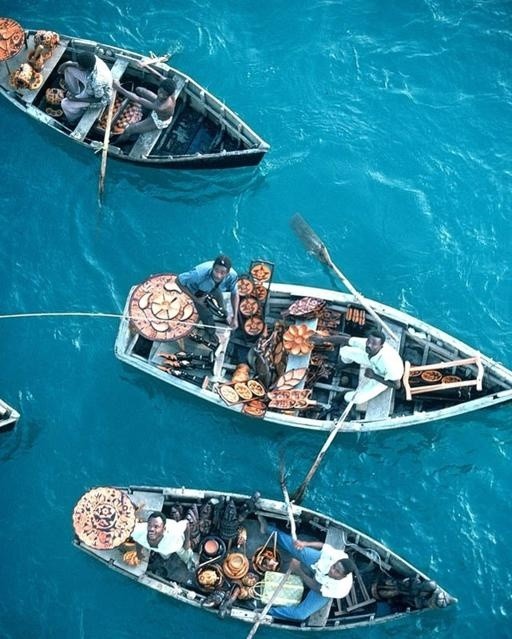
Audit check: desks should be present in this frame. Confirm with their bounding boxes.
[128,273,201,350]
[0,17,28,74]
[72,487,137,550]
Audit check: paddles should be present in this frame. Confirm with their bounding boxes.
[292,392,359,507]
[279,467,299,543]
[289,213,398,342]
[99,88,118,193]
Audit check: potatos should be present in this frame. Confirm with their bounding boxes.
[100,93,143,133]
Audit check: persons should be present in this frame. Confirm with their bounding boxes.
[127,504,196,573]
[176,254,239,344]
[259,514,354,621]
[109,79,176,145]
[57,51,113,125]
[324,331,404,406]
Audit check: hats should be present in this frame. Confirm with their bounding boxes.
[215,255,231,269]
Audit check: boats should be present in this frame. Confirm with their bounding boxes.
[72,487,459,632]
[114,284,512,432]
[0,29,271,170]
